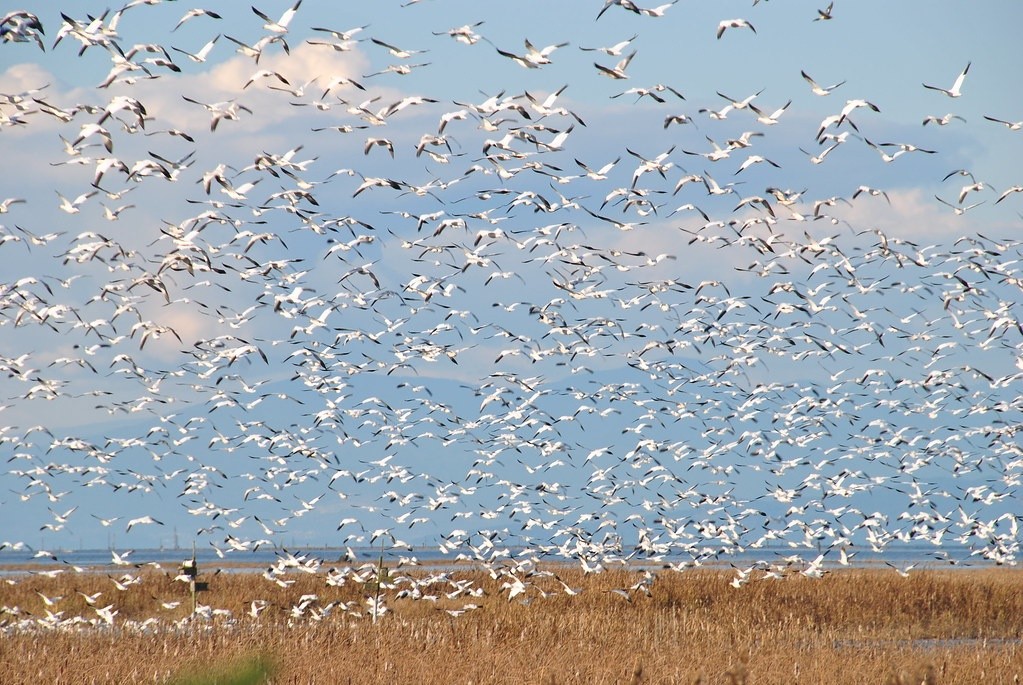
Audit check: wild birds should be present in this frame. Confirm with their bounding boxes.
[0,0,1023,635]
